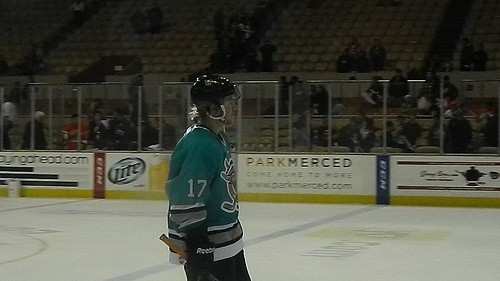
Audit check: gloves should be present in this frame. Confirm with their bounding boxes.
[186,224,214,272]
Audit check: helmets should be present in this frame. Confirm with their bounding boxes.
[190,75,241,110]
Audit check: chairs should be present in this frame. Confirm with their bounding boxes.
[0,0,500,154]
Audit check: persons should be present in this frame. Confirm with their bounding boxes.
[0,0,499,152]
[165,75,252,281]
